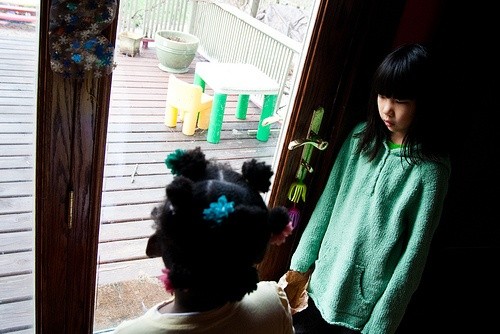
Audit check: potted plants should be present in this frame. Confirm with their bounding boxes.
[118,7,146,58]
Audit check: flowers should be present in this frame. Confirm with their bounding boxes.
[270,221,294,247]
[202,195,237,223]
[165,149,190,180]
[158,268,177,294]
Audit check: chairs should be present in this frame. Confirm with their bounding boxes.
[164,73,215,136]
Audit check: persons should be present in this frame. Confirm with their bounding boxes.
[111,146,296,334]
[289,44,453,334]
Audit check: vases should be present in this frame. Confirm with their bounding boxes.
[154,30,200,74]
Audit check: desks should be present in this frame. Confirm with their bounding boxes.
[194,62,280,143]
